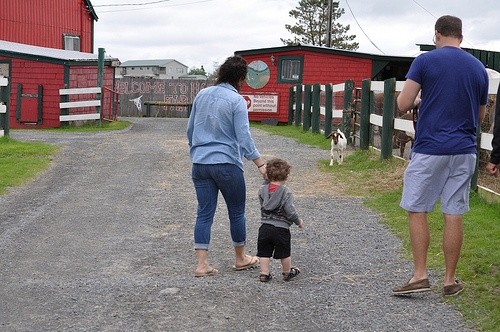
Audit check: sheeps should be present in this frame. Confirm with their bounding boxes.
[325,128,348,168]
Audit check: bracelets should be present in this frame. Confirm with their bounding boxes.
[258,164,264,168]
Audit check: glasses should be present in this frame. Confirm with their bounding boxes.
[433,35,437,44]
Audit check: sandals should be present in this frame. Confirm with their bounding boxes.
[259,272,272,282]
[282,267,301,281]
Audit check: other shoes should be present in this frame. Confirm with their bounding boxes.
[444,279,464,297]
[391,276,431,294]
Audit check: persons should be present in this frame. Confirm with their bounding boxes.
[258,158,304,282]
[187,55,271,277]
[486,82,500,176]
[392,15,489,297]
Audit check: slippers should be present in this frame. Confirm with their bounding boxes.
[194,267,218,277]
[236,256,260,270]
